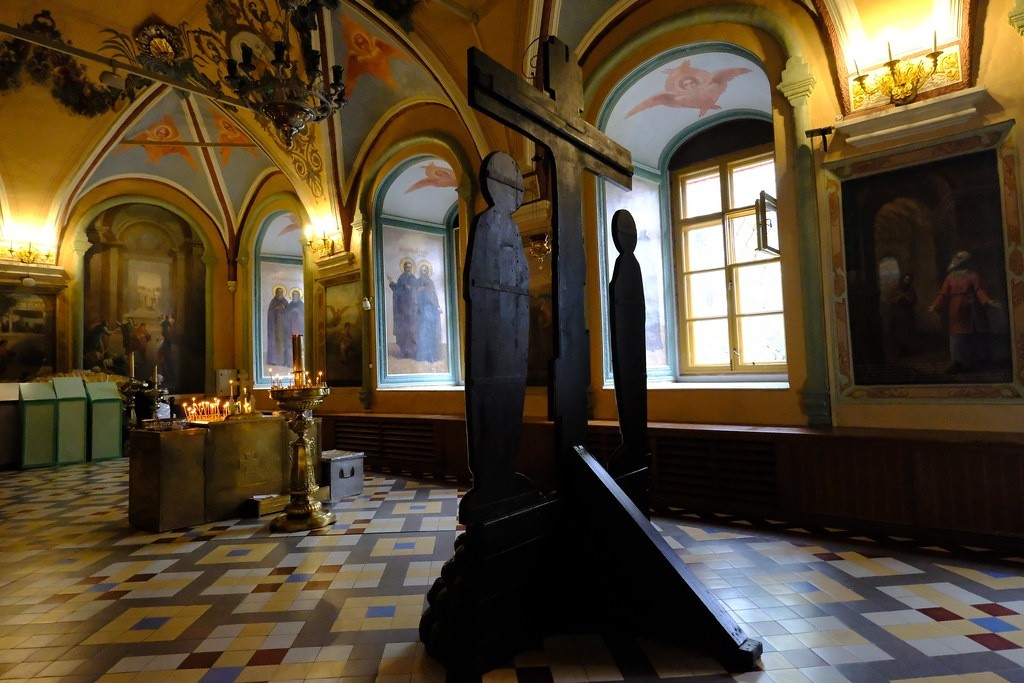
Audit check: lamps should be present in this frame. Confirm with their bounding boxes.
[852,29,944,105]
[8,239,51,264]
[224,0,349,149]
[305,214,329,251]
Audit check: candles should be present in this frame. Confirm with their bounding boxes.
[269,367,323,388]
[230,379,234,396]
[152,364,158,383]
[244,388,248,413]
[129,351,135,377]
[182,397,242,421]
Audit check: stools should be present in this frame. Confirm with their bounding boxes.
[321,449,366,501]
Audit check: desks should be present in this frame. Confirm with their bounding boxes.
[127,427,207,530]
[181,415,323,523]
